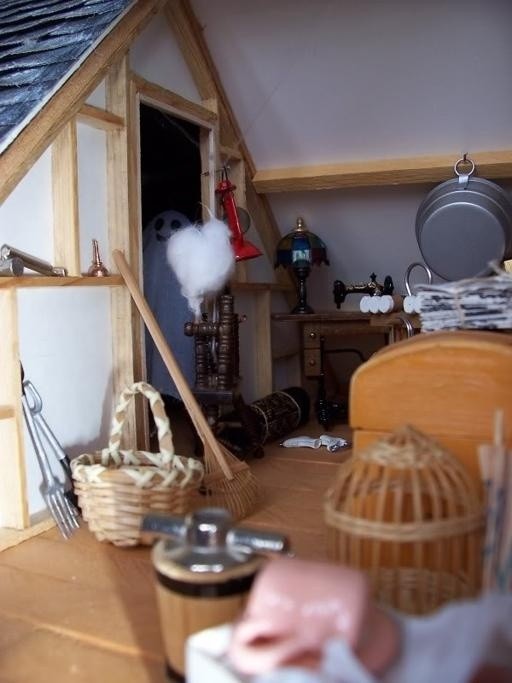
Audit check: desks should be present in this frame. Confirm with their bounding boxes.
[271,312,421,378]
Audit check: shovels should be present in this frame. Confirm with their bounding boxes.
[23,379,82,515]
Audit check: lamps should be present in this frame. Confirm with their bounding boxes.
[273,218,331,314]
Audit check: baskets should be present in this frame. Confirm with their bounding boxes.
[67,382,207,549]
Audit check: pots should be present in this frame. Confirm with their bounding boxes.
[416,157,512,284]
